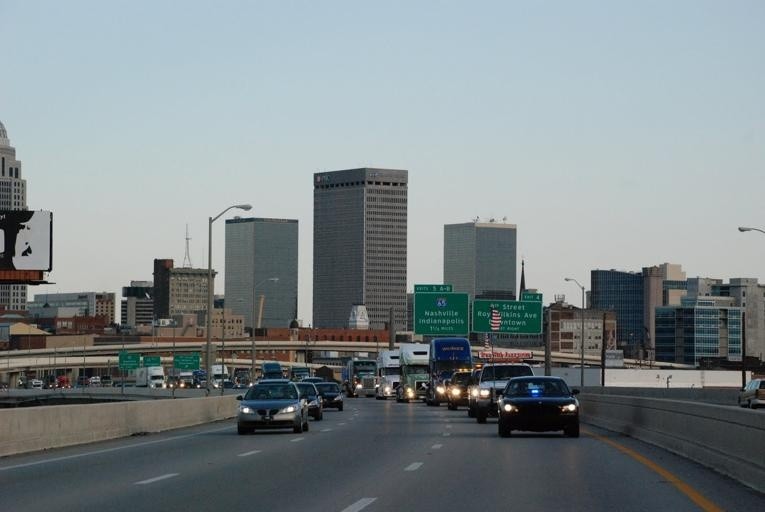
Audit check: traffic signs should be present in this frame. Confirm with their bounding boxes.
[472,290,545,335]
[142,356,161,367]
[413,283,471,338]
[174,354,201,370]
[119,353,141,369]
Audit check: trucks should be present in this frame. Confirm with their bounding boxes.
[347,337,473,408]
[17,364,229,390]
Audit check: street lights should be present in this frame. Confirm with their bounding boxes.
[206,204,252,394]
[565,277,586,388]
[249,277,282,339]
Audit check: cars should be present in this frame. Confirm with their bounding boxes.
[229,361,343,436]
[443,362,581,441]
[737,379,765,409]
